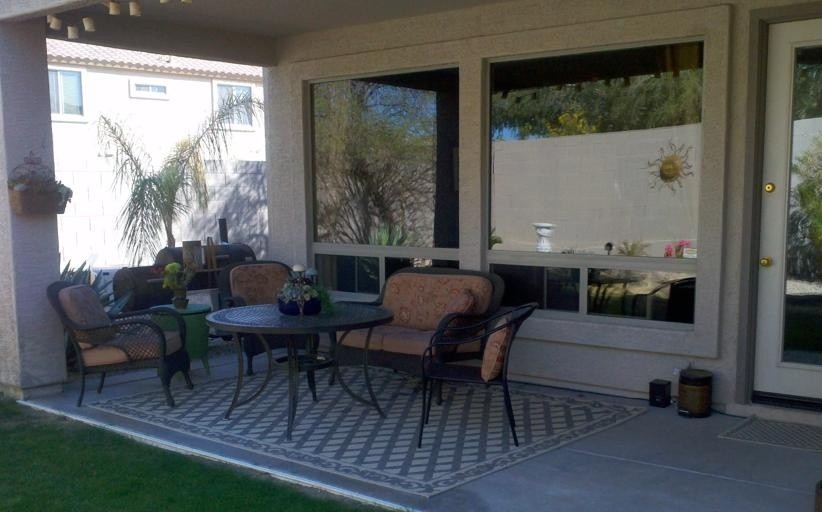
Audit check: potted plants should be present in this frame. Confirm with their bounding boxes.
[6,164,71,217]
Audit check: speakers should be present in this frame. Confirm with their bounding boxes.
[649,379,671,408]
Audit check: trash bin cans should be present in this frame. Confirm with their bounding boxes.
[677,368,713,418]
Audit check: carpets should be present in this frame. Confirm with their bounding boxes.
[714,416,822,454]
[16,343,658,502]
